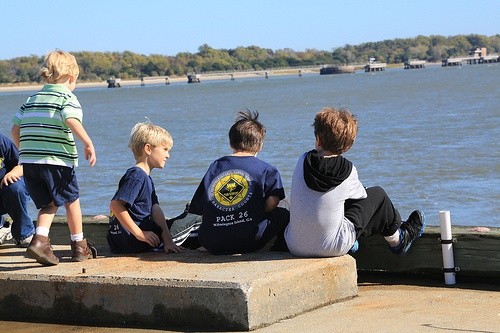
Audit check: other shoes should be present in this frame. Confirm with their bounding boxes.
[16,234,33,247]
[0,223,13,245]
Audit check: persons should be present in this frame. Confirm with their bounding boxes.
[0,133,35,249]
[107,122,201,255]
[283,107,427,258]
[188,109,290,256]
[10,51,97,267]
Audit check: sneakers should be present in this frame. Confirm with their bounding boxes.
[27,233,59,266]
[388,209,426,256]
[71,238,97,262]
